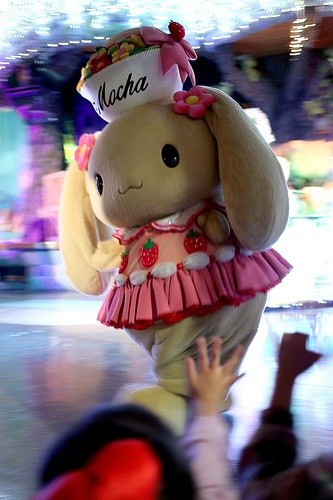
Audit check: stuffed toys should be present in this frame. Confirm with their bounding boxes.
[55,19,292,439]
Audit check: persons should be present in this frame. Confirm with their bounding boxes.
[233,331,331,499]
[27,334,240,500]
